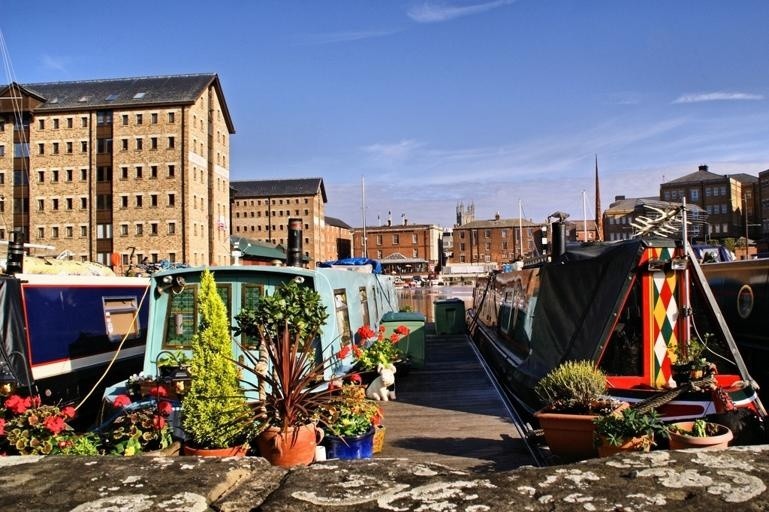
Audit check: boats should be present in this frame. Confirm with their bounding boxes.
[102,258,398,410]
[1,226,153,437]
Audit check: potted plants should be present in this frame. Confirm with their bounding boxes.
[184,268,385,470]
[533,333,734,458]
[154,350,193,377]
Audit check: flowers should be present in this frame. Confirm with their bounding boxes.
[0,384,181,456]
[352,324,416,368]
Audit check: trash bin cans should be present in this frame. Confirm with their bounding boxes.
[433,299,467,334]
[379,311,426,370]
